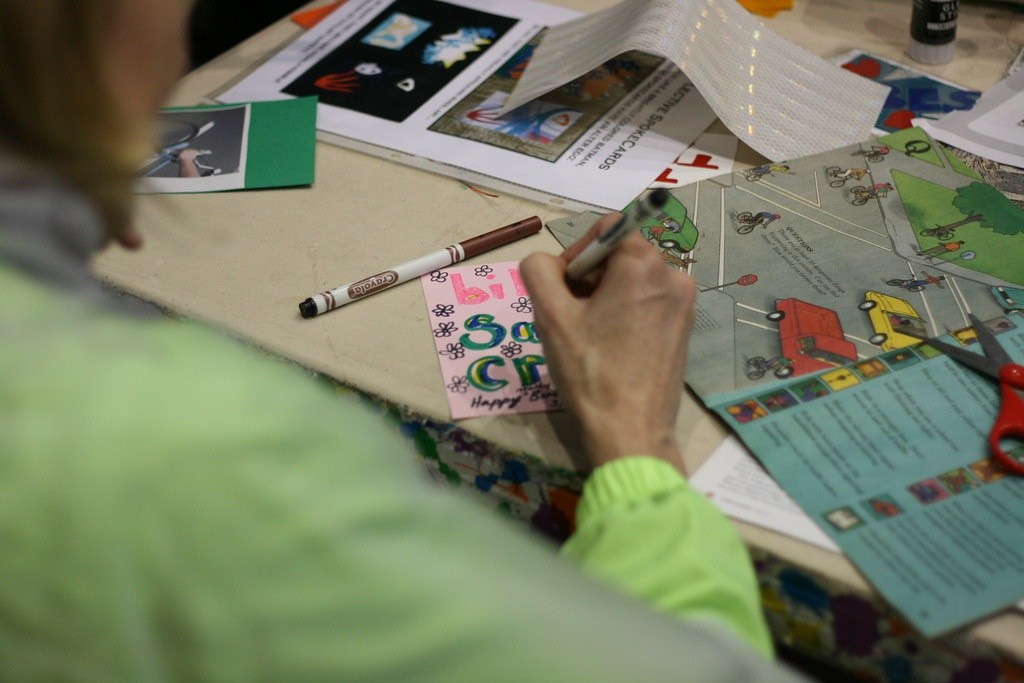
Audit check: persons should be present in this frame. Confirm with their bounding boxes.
[178,148,201,178]
[0,0,804,682]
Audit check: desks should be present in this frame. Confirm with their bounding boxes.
[80,0,1024,683]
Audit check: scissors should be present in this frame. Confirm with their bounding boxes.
[923,309,1024,479]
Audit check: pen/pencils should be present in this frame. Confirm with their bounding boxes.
[299,212,544,327]
[565,190,673,287]
